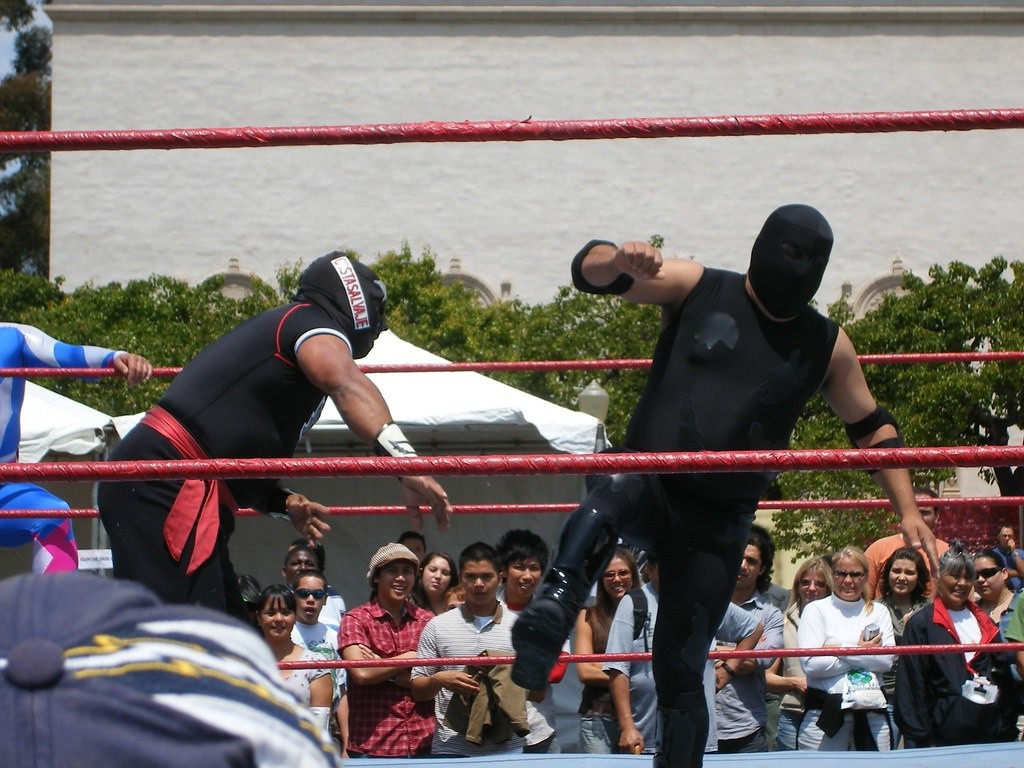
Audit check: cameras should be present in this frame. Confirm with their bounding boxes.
[864,623,879,642]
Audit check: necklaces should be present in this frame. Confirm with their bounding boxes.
[984,590,1012,615]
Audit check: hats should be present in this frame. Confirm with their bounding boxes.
[366,542,420,587]
[1,570,343,768]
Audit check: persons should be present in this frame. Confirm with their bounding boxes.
[97,251,450,621]
[232,512,1024,758]
[0,323,152,576]
[0,574,339,768]
[510,204,943,768]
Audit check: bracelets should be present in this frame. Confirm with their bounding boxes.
[722,662,735,677]
[1012,552,1019,559]
[372,421,419,482]
[270,485,293,513]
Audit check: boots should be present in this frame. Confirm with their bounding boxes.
[512,508,617,691]
[653,703,710,768]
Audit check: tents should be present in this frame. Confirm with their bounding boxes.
[19,329,614,612]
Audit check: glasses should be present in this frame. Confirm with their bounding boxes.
[974,567,1000,579]
[832,569,866,580]
[797,578,827,589]
[294,589,326,600]
[602,569,633,583]
